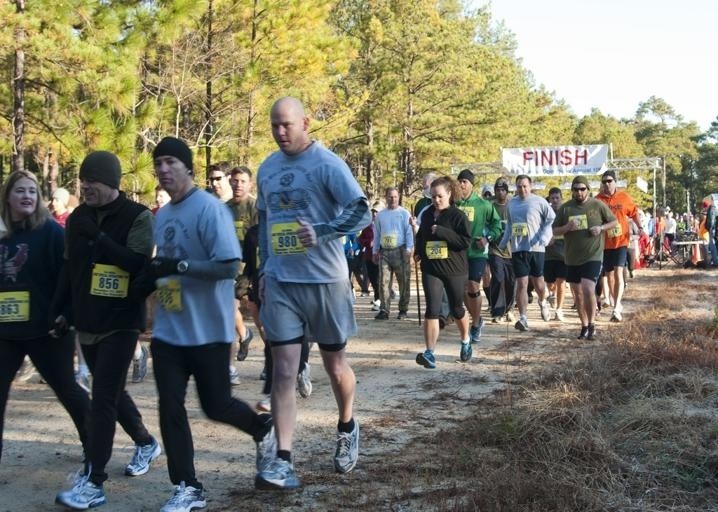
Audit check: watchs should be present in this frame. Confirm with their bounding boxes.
[177,259,191,275]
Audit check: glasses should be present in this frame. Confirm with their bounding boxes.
[209,176,222,180]
[573,188,586,192]
[602,179,613,184]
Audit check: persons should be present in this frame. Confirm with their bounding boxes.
[243,224,311,411]
[50,151,161,509]
[131,341,149,382]
[544,187,567,321]
[360,200,384,311]
[552,177,618,340]
[344,230,368,298]
[206,162,241,385]
[49,188,70,226]
[1,171,91,481]
[254,97,373,490]
[224,167,259,361]
[416,176,472,369]
[373,186,413,319]
[646,201,718,267]
[152,137,276,512]
[499,174,555,331]
[594,171,646,322]
[485,177,515,323]
[456,169,503,343]
[153,186,170,212]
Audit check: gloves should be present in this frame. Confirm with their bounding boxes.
[149,256,180,278]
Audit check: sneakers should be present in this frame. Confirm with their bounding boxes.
[255,424,278,471]
[361,292,369,296]
[236,329,253,360]
[375,311,389,320]
[416,350,436,369]
[587,323,595,340]
[55,478,105,509]
[125,438,161,475]
[297,362,312,399]
[470,325,480,342]
[132,345,148,382]
[160,482,207,512]
[549,297,556,308]
[515,318,528,331]
[255,459,301,491]
[333,418,360,474]
[601,299,610,308]
[609,309,622,322]
[506,313,515,322]
[256,397,271,412]
[479,317,483,336]
[229,366,240,385]
[372,300,380,311]
[538,299,551,322]
[492,316,503,323]
[460,334,472,361]
[554,311,564,321]
[75,374,91,394]
[397,311,407,319]
[577,326,588,340]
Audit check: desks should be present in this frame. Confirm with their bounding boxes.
[671,239,705,246]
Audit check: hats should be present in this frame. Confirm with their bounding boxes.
[494,181,508,192]
[52,188,70,206]
[152,137,193,170]
[370,201,386,213]
[457,169,475,186]
[79,150,121,189]
[571,176,590,190]
